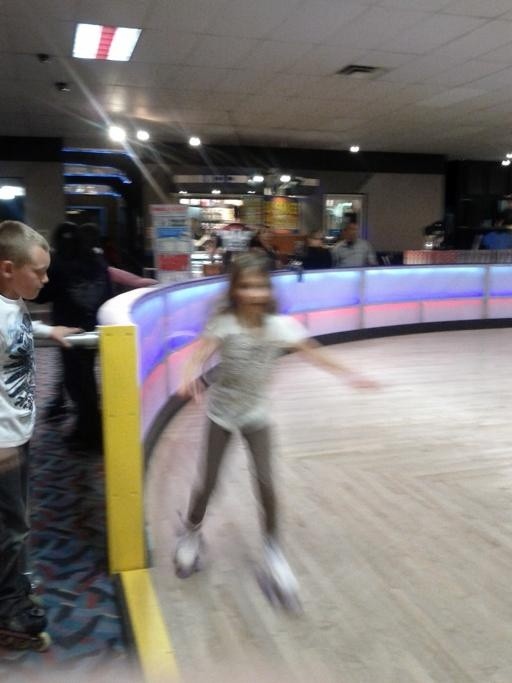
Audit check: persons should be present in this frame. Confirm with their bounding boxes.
[0,220,86,649]
[479,211,512,250]
[331,219,379,269]
[32,222,158,455]
[172,254,382,610]
[250,227,286,272]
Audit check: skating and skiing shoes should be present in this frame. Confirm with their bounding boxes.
[0,605,52,652]
[256,553,296,605]
[174,527,203,576]
